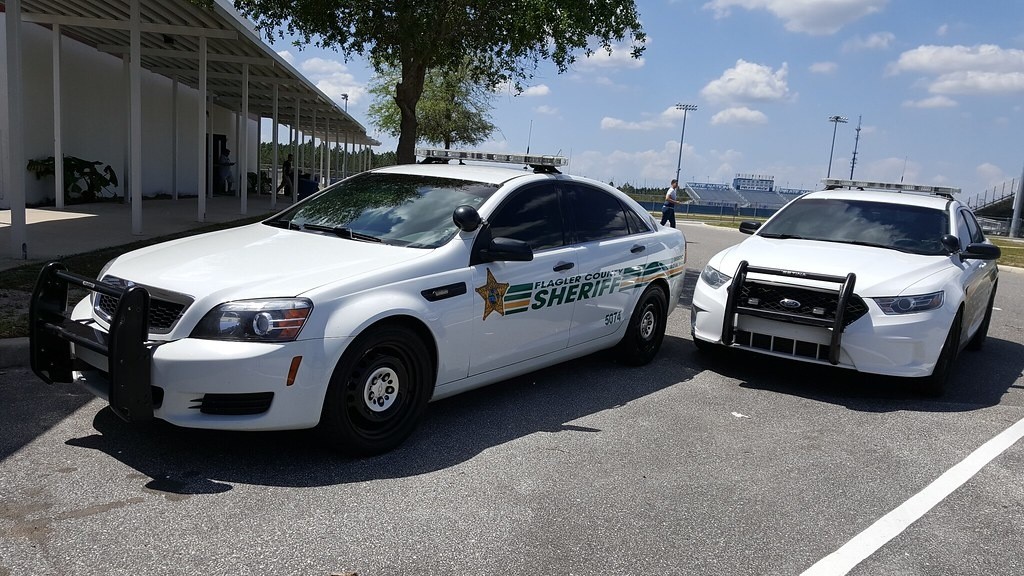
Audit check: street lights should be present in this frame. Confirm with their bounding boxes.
[671,102,697,193]
[341,92,348,112]
[826,113,848,181]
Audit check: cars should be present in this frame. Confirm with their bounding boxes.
[686,179,1001,397]
[23,163,686,453]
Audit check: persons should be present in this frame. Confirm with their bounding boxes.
[660,179,680,228]
[219,149,235,193]
[276,154,294,198]
